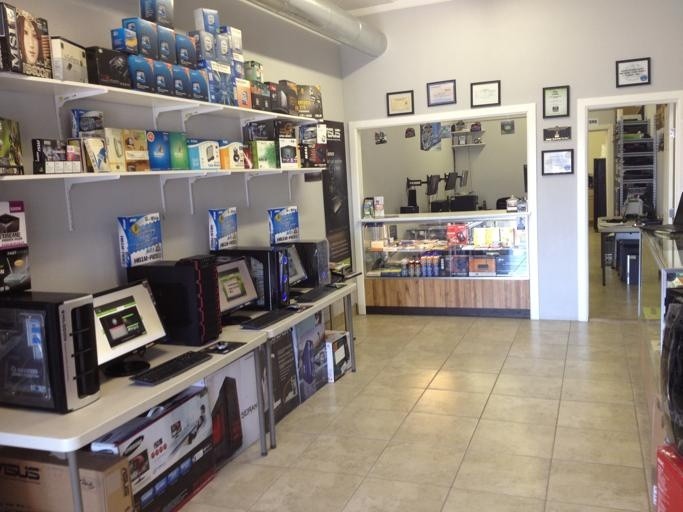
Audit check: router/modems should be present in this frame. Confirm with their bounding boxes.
[4,255,30,286]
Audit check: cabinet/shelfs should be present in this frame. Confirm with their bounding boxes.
[614,118,657,218]
[0,71,327,184]
[361,209,530,320]
[450,130,484,150]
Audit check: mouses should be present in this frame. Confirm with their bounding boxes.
[328,283,337,287]
[216,341,228,350]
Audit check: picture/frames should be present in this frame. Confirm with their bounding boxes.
[616,57,650,87]
[427,80,456,106]
[543,86,569,118]
[471,80,501,108]
[387,90,414,117]
[542,149,573,175]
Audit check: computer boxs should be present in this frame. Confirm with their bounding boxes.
[0,291,101,415]
[127,239,328,346]
[211,377,242,464]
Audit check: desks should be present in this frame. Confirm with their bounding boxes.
[1,283,358,511]
[597,216,662,286]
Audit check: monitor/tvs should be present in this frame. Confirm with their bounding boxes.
[129,448,150,486]
[440,172,458,190]
[91,277,166,375]
[170,420,182,439]
[407,174,440,195]
[140,442,212,509]
[444,169,468,186]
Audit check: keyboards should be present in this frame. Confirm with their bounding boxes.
[129,351,212,386]
